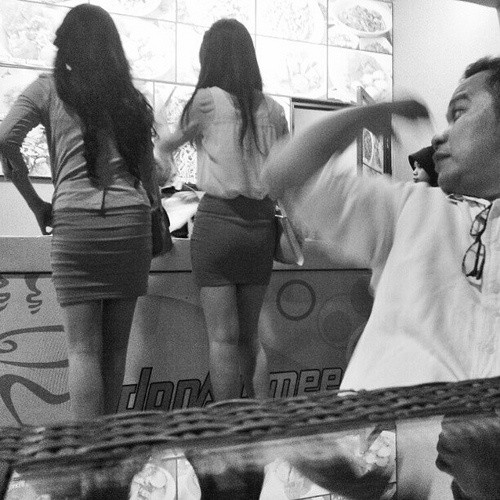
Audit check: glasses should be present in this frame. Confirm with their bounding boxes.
[461,200,494,280]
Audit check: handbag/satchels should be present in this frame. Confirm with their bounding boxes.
[274,216,304,267]
[151,205,173,260]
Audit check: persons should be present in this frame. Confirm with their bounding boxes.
[153,15,299,417]
[250,52,500,499]
[0,3,171,429]
[407,141,440,193]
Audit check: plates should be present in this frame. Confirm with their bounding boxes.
[105,0,161,65]
[251,0,392,96]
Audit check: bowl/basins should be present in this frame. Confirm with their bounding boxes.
[330,0,391,38]
